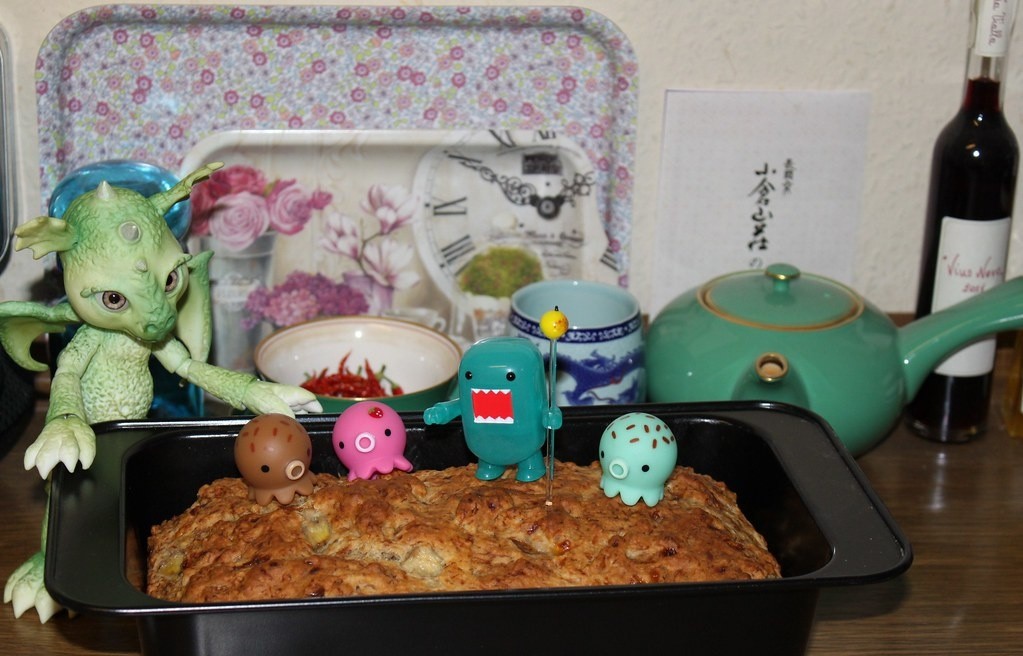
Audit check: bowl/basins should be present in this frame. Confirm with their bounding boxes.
[256,314,463,414]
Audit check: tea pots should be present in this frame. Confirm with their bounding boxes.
[645,263,1023,459]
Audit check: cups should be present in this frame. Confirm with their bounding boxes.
[507,278,645,407]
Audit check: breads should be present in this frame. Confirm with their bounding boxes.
[146,455,782,602]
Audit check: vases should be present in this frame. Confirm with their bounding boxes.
[207,231,270,368]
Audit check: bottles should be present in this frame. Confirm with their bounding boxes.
[908,0,1019,443]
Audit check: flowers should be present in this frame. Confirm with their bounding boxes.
[183,167,414,336]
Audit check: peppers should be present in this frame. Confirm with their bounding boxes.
[302,351,406,398]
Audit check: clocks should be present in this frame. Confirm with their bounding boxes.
[412,125,608,315]
